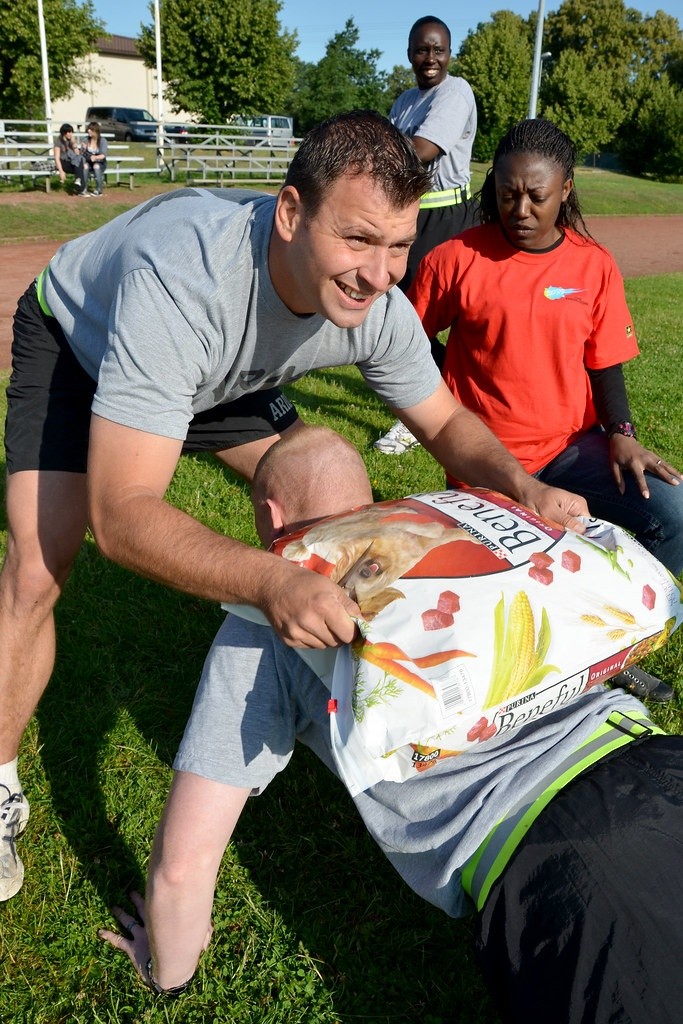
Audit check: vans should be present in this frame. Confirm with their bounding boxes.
[86,107,161,141]
[228,116,292,148]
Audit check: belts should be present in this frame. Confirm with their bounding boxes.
[419,183,472,209]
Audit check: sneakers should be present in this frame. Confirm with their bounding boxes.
[0,784,31,903]
[373,419,421,455]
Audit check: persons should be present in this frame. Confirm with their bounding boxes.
[405,119,683,701]
[374,16,477,455]
[98,424,683,1024]
[0,109,591,903]
[54,122,107,197]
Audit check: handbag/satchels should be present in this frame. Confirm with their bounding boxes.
[30,161,56,171]
[83,151,106,168]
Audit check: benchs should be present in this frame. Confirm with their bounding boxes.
[0,119,304,192]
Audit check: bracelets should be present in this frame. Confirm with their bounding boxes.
[145,958,187,998]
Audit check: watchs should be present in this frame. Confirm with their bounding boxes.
[607,421,636,439]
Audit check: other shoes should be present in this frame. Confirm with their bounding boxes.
[90,191,103,197]
[78,192,90,198]
[74,178,81,186]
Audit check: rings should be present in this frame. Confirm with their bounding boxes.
[128,920,138,932]
[656,460,662,465]
